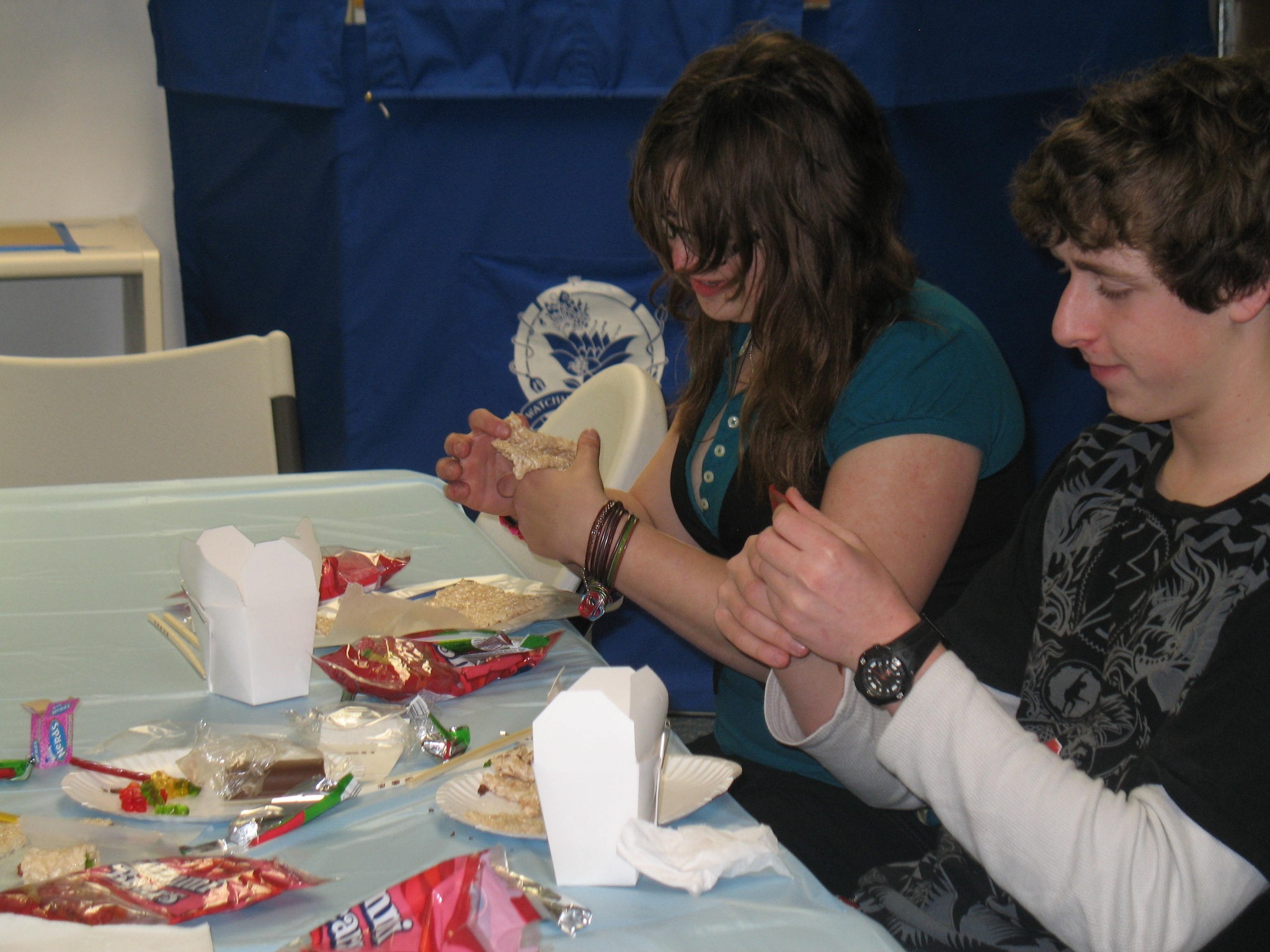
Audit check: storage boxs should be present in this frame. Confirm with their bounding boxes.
[531,664,668,887]
[177,516,322,707]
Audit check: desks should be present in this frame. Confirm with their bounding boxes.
[1,216,162,355]
[0,470,911,952]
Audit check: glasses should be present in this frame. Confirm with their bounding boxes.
[657,217,783,266]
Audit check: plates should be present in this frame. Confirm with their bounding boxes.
[60,745,325,821]
[434,751,742,840]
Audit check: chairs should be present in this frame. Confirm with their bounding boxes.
[0,331,303,487]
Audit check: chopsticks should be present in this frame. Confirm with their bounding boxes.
[350,728,530,794]
[149,612,207,678]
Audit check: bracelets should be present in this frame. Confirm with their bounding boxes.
[579,499,639,627]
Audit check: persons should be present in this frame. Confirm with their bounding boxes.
[435,31,1024,884]
[708,55,1269,952]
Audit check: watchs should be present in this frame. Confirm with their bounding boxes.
[852,615,948,707]
